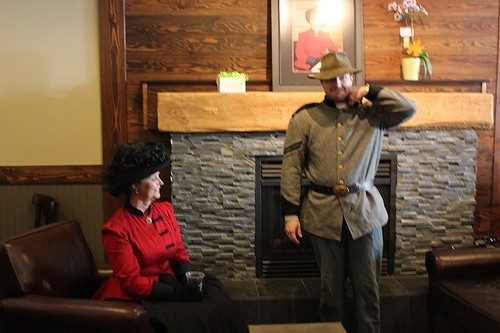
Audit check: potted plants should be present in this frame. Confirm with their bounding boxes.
[217,71,248,93]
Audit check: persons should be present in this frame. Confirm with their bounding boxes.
[91,141,251,333]
[293,6,341,70]
[280,51,416,333]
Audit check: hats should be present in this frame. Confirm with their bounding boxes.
[102,140,170,197]
[308,51,364,81]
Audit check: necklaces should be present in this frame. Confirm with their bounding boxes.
[145,217,152,224]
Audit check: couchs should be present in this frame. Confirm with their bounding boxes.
[425,248,500,333]
[0,219,153,333]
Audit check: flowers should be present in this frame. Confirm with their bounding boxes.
[388,0,433,80]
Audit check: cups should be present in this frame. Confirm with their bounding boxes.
[185,271,205,292]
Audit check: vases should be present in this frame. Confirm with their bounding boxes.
[401,58,421,81]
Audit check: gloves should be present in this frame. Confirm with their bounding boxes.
[173,286,203,302]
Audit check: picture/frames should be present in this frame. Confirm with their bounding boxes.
[270,0,364,91]
